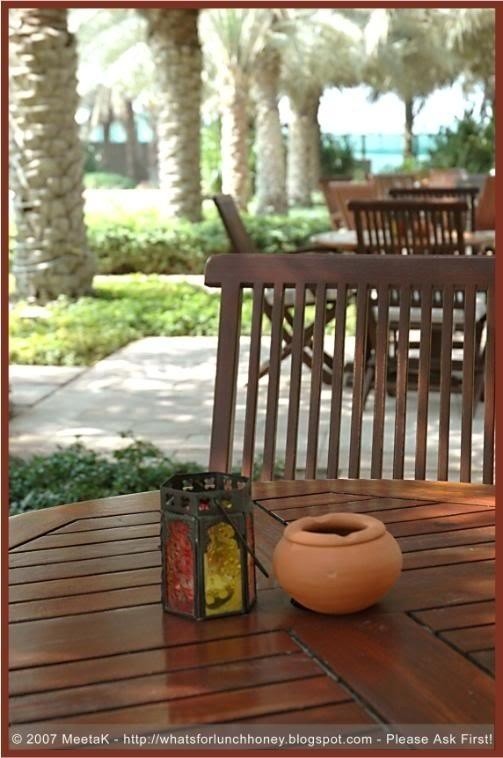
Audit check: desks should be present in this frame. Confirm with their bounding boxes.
[6,481,494,748]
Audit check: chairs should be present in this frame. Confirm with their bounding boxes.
[204,165,495,485]
